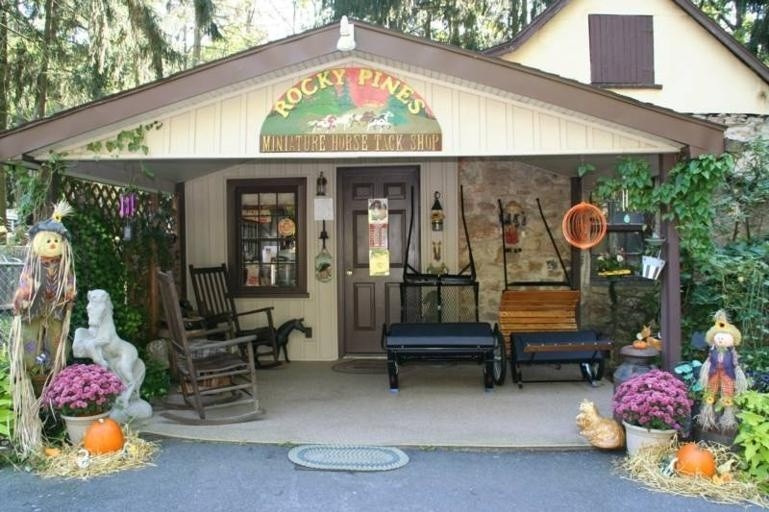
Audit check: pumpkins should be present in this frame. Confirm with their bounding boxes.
[83,418,124,455]
[674,443,715,479]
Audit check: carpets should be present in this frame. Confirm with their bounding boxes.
[331,358,418,377]
[288,444,410,472]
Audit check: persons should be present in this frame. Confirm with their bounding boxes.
[693,307,748,429]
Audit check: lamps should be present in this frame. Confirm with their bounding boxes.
[317,170,328,195]
[337,15,355,51]
[430,191,445,231]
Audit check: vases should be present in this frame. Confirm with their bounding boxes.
[611,345,661,392]
[59,412,112,447]
[621,422,678,460]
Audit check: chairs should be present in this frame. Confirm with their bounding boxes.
[156,261,285,424]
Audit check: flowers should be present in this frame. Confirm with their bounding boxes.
[610,370,694,433]
[43,364,128,416]
